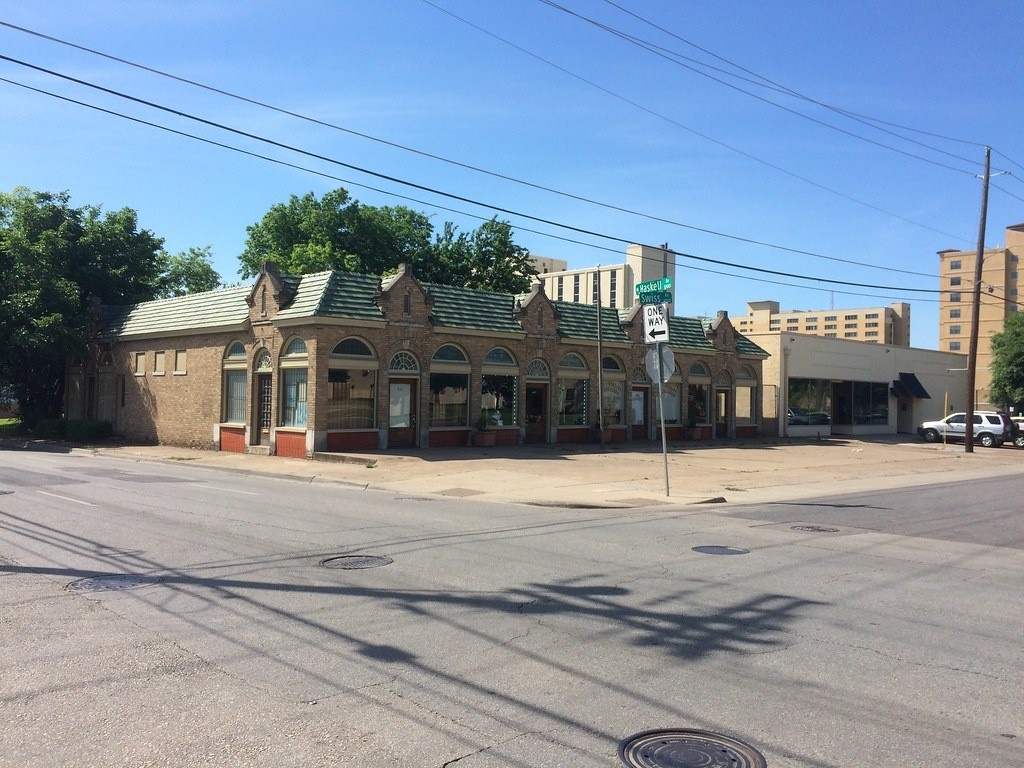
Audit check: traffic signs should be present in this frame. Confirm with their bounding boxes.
[642,302,669,345]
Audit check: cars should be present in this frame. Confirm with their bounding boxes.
[787,406,830,426]
[1009,417,1024,450]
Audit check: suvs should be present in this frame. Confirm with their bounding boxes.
[917,410,1016,448]
